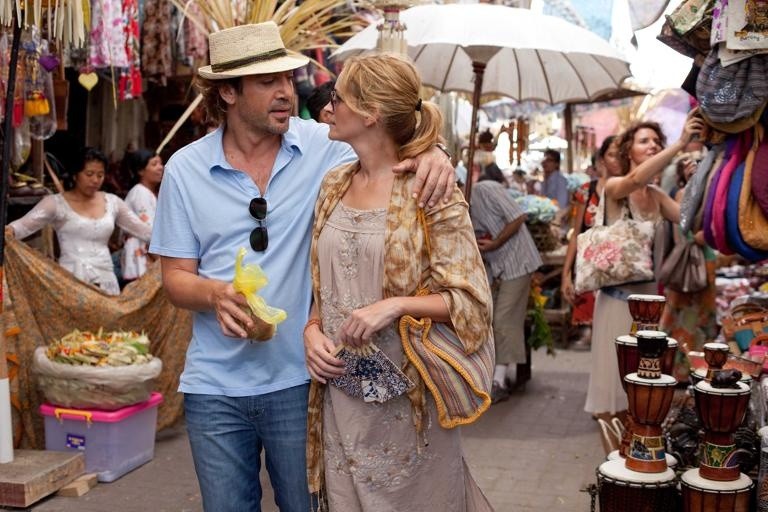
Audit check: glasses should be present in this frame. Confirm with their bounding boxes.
[249,197,269,252]
[329,89,342,108]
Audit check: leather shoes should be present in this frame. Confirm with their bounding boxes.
[491,378,508,405]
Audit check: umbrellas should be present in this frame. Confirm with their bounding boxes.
[562,81,649,176]
[329,1,634,215]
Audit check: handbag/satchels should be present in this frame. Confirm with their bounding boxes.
[658,0,768,260]
[397,195,496,431]
[573,177,657,294]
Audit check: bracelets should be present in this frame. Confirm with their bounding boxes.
[303,319,322,330]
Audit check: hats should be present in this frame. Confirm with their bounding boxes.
[197,18,311,80]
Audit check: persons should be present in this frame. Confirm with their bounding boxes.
[149,21,455,512]
[121,150,162,279]
[5,148,152,296]
[583,105,704,427]
[540,149,567,208]
[666,151,704,255]
[561,135,621,325]
[512,169,542,196]
[455,169,544,403]
[302,53,496,512]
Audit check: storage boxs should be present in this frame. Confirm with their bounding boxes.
[40,393,165,482]
